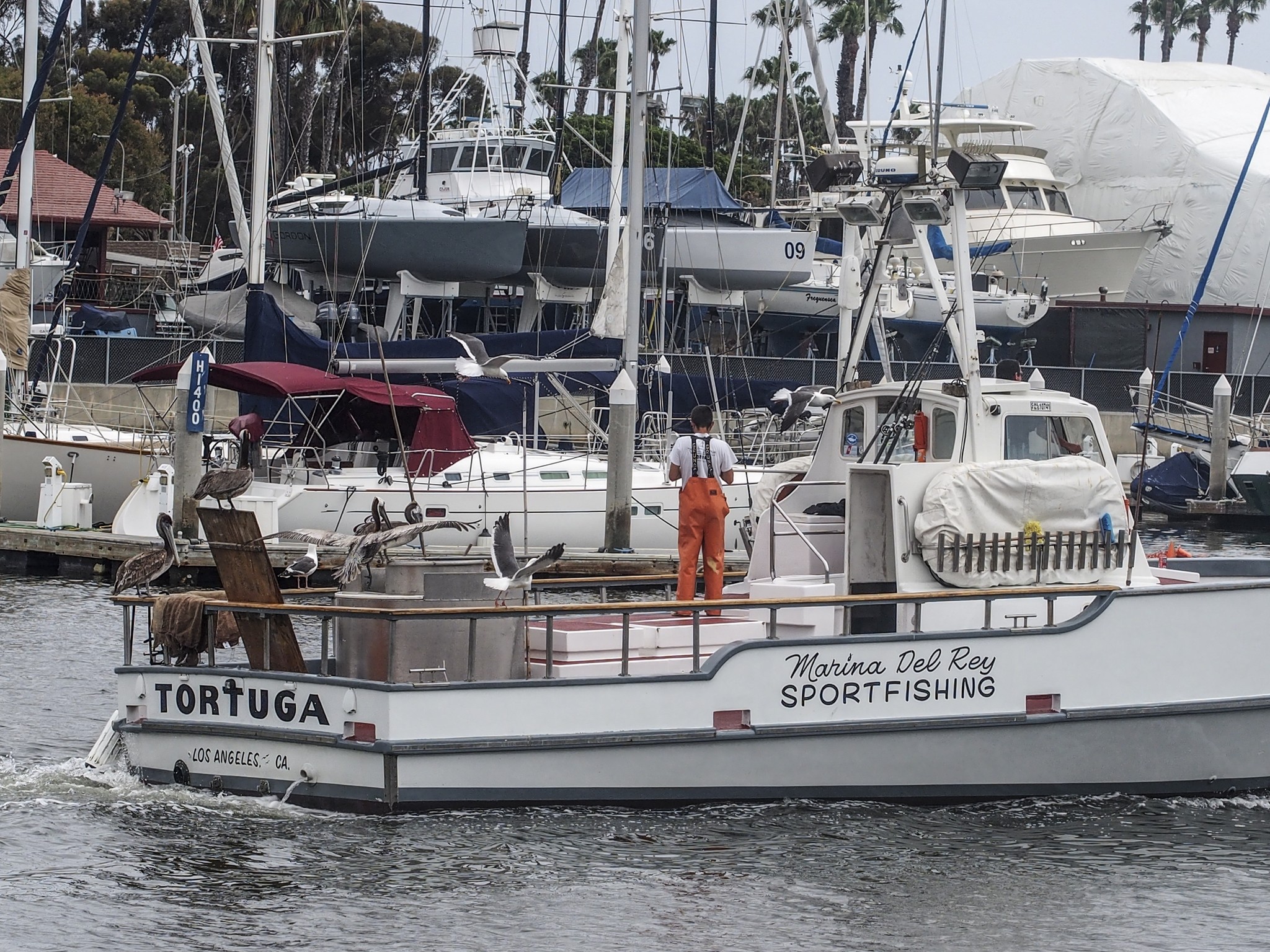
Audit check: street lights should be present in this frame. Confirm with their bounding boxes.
[91,132,125,192]
[135,70,225,243]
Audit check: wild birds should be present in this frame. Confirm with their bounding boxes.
[107,513,182,600]
[769,383,843,436]
[482,509,569,609]
[238,497,483,592]
[188,428,254,514]
[443,329,545,385]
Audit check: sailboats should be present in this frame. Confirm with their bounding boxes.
[0,0,1270,812]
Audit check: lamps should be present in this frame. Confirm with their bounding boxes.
[835,195,887,227]
[802,151,864,194]
[902,193,952,227]
[945,149,1009,187]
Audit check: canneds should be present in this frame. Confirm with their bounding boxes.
[917,449,926,462]
[1158,554,1167,568]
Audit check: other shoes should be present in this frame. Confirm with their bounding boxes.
[671,611,690,617]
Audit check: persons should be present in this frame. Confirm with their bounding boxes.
[667,405,738,618]
[995,358,1082,458]
